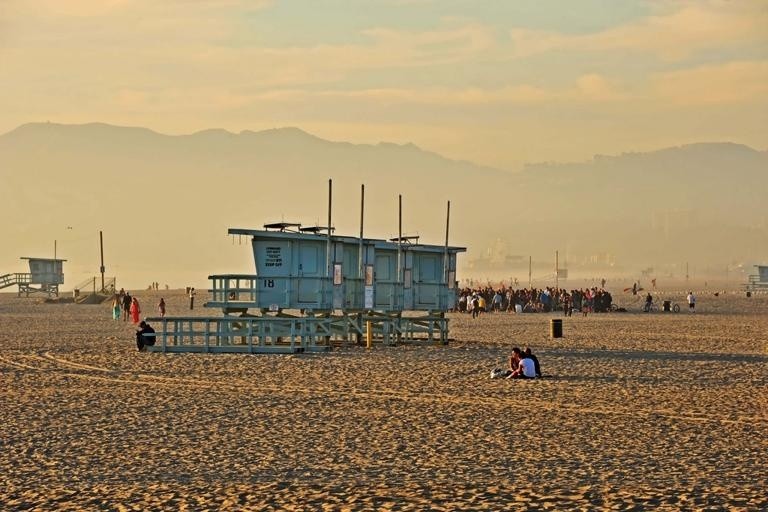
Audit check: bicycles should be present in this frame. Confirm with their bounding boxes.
[661,303,679,313]
[608,303,617,311]
[644,301,658,312]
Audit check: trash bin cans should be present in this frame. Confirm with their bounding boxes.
[747,292,750,297]
[550,318,562,338]
[663,301,671,312]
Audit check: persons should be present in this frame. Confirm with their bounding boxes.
[450,277,612,319]
[110,284,141,325]
[189,288,196,310]
[643,293,652,312]
[504,347,541,379]
[687,292,696,313]
[159,297,165,317]
[135,321,156,352]
